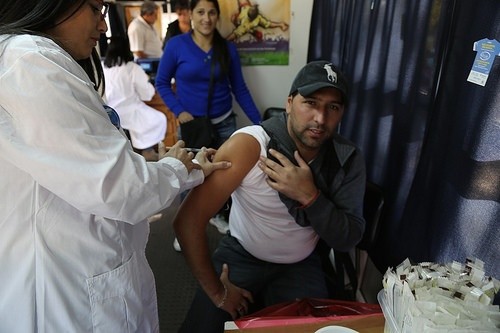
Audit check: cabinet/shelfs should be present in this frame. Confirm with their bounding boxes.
[146,85,181,151]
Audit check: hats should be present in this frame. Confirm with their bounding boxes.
[289,61,347,102]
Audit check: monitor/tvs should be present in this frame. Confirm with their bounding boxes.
[138,59,160,75]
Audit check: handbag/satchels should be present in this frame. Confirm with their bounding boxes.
[180,118,219,157]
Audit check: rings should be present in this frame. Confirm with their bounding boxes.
[238,307,243,313]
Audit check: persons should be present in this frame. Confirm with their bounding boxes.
[128,0,164,62]
[155,0,263,252]
[97,30,167,154]
[0,0,232,333]
[162,0,192,51]
[173,61,367,333]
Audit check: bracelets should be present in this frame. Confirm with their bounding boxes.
[295,190,320,209]
[216,285,228,308]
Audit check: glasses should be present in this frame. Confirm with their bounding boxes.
[96,0,109,19]
[102,106,120,131]
